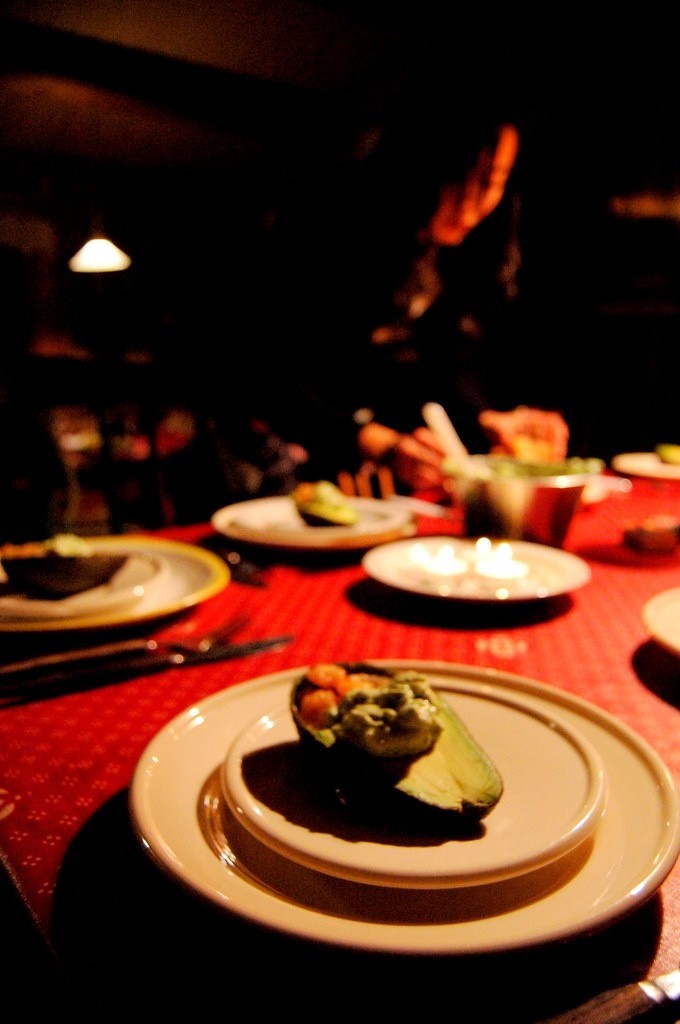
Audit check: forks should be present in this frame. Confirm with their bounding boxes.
[8,614,252,678]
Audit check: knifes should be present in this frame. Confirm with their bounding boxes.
[9,636,293,706]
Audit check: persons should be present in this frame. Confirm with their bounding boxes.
[145,78,573,508]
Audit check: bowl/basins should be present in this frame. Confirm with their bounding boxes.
[459,479,592,548]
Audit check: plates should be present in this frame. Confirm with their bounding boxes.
[213,497,439,553]
[0,537,231,634]
[641,586,680,658]
[232,503,404,538]
[360,535,591,602]
[611,452,680,481]
[130,661,680,955]
[0,553,167,617]
[219,683,605,889]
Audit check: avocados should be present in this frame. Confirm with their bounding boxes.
[1,533,128,598]
[290,663,505,823]
[295,480,359,528]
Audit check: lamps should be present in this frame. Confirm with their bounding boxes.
[67,207,133,275]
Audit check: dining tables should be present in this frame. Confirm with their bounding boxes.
[0,475,680,1024]
